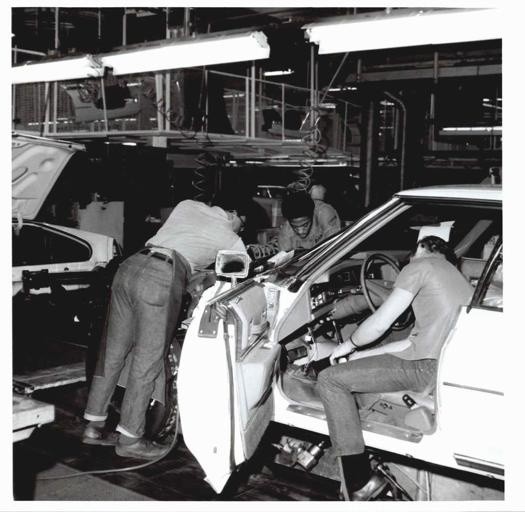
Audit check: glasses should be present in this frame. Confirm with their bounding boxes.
[230,210,247,232]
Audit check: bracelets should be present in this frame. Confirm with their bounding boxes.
[349,335,360,349]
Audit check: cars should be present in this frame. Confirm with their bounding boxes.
[12,123,131,392]
[162,177,504,502]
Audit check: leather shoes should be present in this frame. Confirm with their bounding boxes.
[350,473,388,501]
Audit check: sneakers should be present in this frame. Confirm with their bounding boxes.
[116,435,169,461]
[83,425,118,448]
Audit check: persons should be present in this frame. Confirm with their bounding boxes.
[78,197,258,461]
[312,235,477,501]
[276,192,345,255]
